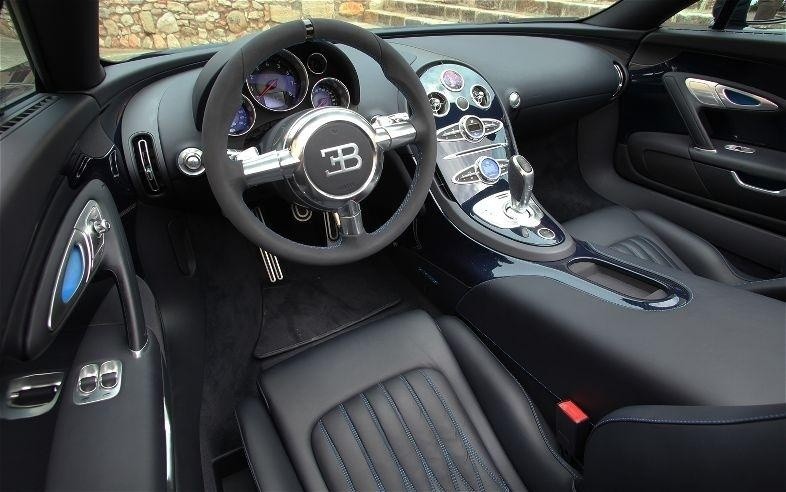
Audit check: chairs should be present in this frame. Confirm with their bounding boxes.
[556,198,785,302]
[231,310,785,492]
